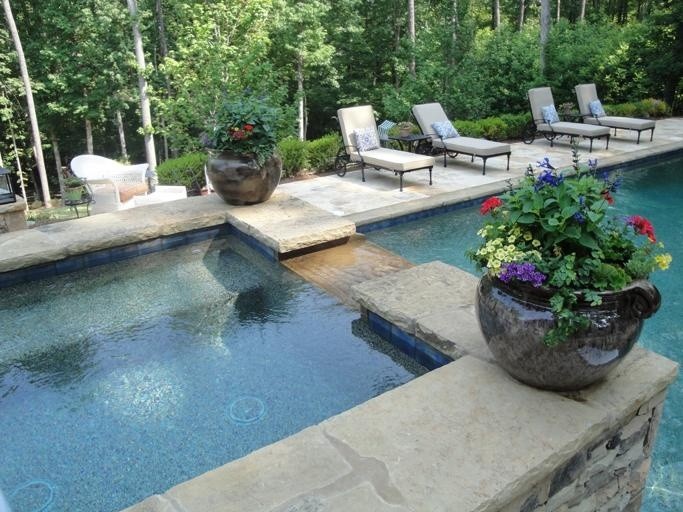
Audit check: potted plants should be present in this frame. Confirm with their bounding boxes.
[63,177,83,200]
[398,121,414,137]
[559,102,576,114]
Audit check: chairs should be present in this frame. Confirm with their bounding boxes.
[70,154,187,214]
[522,86,611,153]
[335,104,435,192]
[410,102,511,175]
[575,83,655,144]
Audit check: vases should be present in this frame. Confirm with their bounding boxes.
[475,270,662,389]
[205,150,282,205]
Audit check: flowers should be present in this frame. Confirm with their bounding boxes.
[465,132,672,286]
[200,85,286,149]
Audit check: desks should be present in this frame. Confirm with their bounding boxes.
[0,189,28,232]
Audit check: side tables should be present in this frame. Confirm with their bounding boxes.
[66,197,91,218]
[559,112,586,122]
[388,134,431,152]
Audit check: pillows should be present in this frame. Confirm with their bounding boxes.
[105,171,150,203]
[541,104,560,123]
[354,126,379,151]
[432,122,460,139]
[589,100,606,117]
